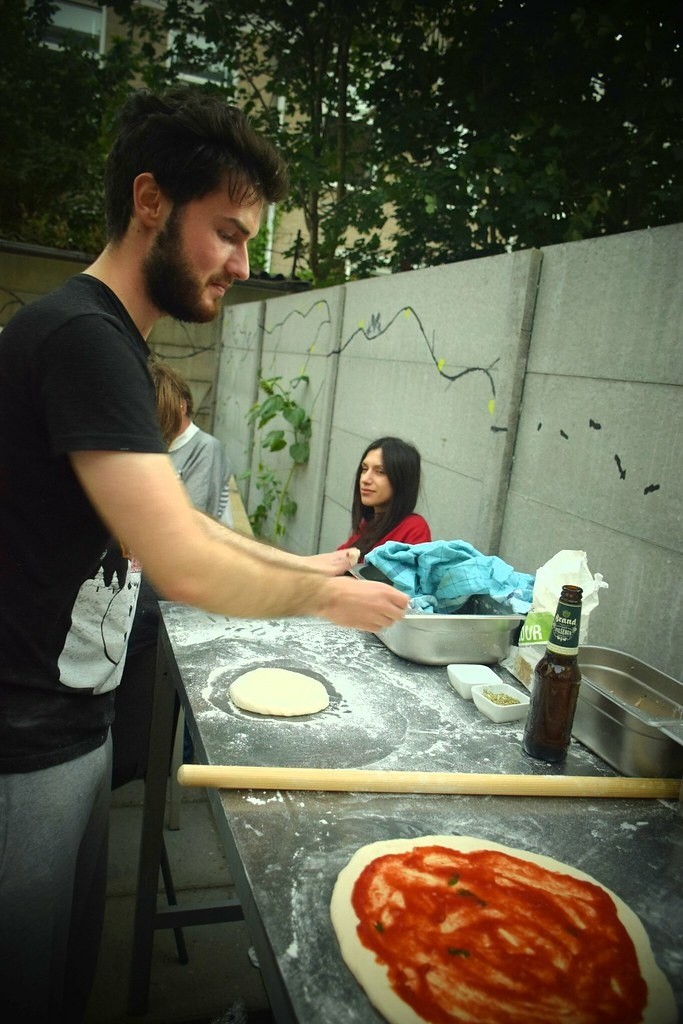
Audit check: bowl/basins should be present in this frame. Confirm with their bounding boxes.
[471,683,531,724]
[446,664,502,700]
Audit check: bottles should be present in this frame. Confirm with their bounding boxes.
[523,584,583,763]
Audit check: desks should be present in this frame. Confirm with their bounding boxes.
[125,598,682,1024]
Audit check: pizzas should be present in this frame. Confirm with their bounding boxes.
[329,834,681,1024]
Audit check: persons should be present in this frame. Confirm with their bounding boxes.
[0,90,412,1021]
[148,359,232,524]
[338,436,430,562]
